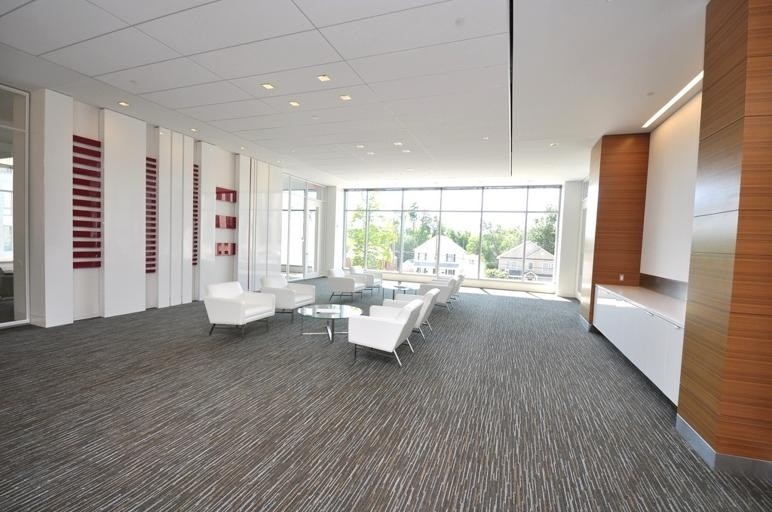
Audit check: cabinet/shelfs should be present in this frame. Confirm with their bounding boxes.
[592,284,687,406]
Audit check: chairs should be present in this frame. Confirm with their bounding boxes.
[203,265,465,368]
